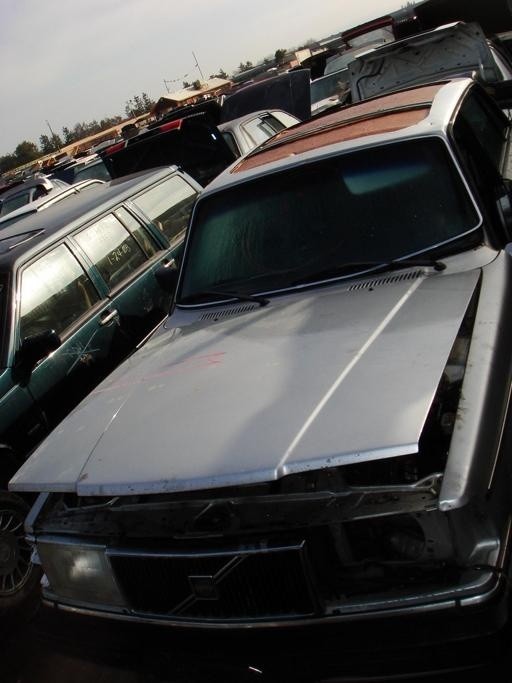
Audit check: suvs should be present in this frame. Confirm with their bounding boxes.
[1,162,206,617]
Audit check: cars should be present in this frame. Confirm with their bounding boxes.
[19,66,512,683]
[1,0,512,187]
